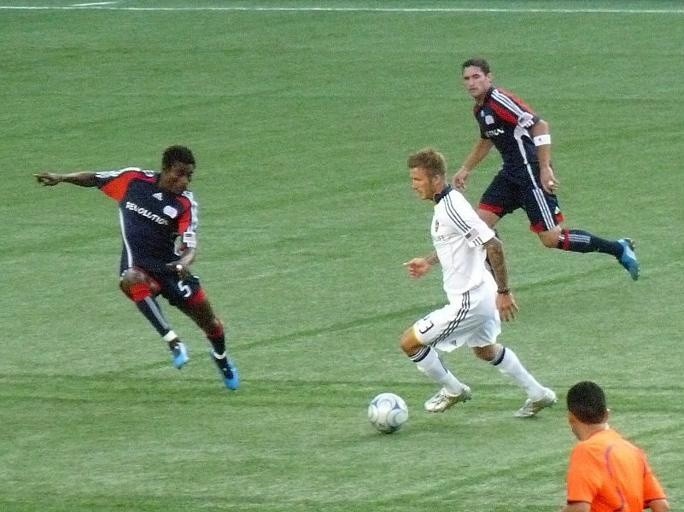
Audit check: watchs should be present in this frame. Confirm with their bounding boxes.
[172,262,182,269]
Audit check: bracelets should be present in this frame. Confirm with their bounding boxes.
[497,288,511,294]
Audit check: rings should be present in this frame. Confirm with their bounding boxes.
[548,180,554,185]
[542,184,546,187]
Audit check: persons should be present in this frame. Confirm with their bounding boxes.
[452,58,642,283]
[34,144,238,390]
[565,379,675,511]
[397,148,558,415]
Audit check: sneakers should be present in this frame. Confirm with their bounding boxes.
[170,341,191,369]
[513,387,559,418]
[211,348,239,390]
[617,237,640,281]
[424,384,472,412]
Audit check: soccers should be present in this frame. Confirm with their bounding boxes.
[366,391,408,433]
